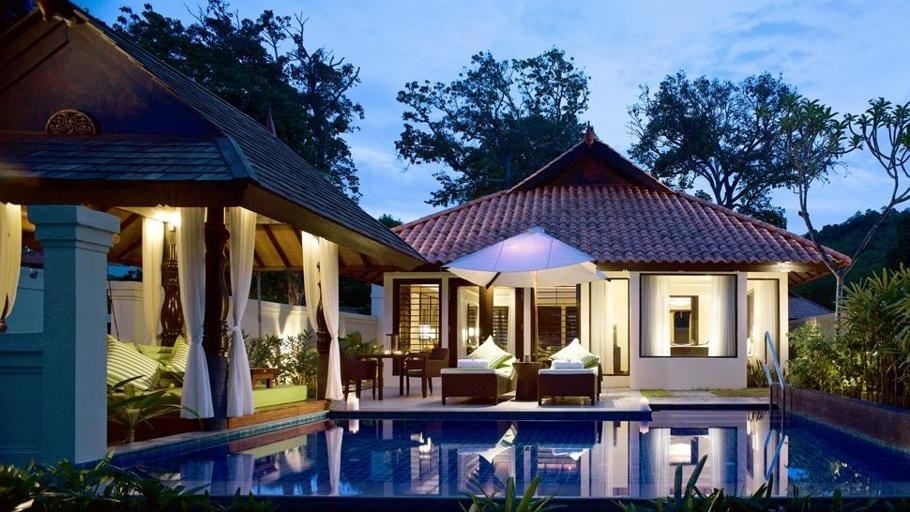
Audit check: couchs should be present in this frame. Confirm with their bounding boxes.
[108,342,182,420]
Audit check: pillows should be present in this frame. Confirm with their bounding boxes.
[476,430,516,463]
[550,337,600,368]
[105,333,190,395]
[552,446,590,461]
[468,335,511,369]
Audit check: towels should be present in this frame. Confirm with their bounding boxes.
[551,359,584,369]
[457,358,489,370]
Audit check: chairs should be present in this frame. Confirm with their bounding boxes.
[339,343,449,401]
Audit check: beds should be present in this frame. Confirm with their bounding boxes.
[537,358,602,406]
[440,355,521,406]
[439,420,603,448]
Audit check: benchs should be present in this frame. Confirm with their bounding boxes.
[250,383,310,410]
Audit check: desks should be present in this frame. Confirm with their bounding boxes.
[248,367,278,390]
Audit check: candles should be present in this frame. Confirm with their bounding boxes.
[640,421,650,434]
[348,419,360,434]
[639,397,649,409]
[346,392,359,409]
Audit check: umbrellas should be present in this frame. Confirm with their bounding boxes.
[438,227,610,364]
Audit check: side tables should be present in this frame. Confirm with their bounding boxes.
[510,361,544,401]
